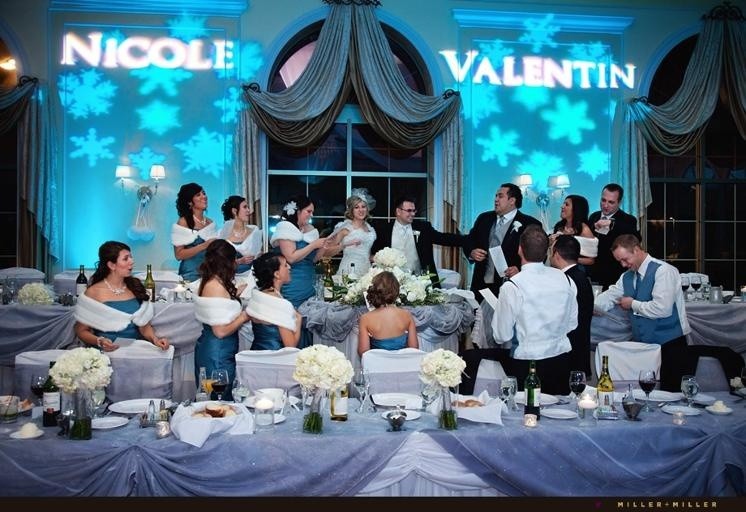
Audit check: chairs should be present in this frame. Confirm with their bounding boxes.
[0,267,46,296]
[14,349,71,407]
[682,274,710,293]
[135,271,185,295]
[360,347,432,396]
[595,340,660,391]
[670,354,742,390]
[100,338,172,401]
[51,272,93,292]
[234,347,302,394]
[436,267,462,290]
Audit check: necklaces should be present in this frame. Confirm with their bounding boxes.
[192,214,206,227]
[232,228,249,240]
[267,285,283,298]
[102,277,128,295]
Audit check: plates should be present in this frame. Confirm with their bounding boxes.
[705,407,733,415]
[91,416,130,430]
[253,412,286,425]
[107,398,172,415]
[9,430,44,440]
[540,407,579,420]
[515,391,559,407]
[661,404,702,417]
[245,395,300,410]
[381,409,421,421]
[631,389,682,403]
[372,393,423,408]
[692,395,716,406]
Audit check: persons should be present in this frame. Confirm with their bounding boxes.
[220,196,262,276]
[270,196,338,349]
[194,240,252,402]
[490,224,579,395]
[357,272,419,357]
[171,183,218,283]
[464,183,542,348]
[594,234,692,393]
[548,234,591,396]
[74,241,170,352]
[371,196,467,289]
[589,184,642,292]
[325,188,377,277]
[545,196,599,278]
[246,252,302,350]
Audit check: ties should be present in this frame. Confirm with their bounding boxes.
[401,227,410,239]
[636,270,641,292]
[600,215,608,221]
[498,215,505,224]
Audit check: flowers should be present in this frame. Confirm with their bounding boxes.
[419,347,467,430]
[293,345,355,435]
[319,247,446,307]
[15,282,54,306]
[50,347,113,440]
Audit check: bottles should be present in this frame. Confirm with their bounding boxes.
[524,360,541,420]
[42,362,61,427]
[145,264,155,303]
[196,367,209,408]
[331,366,349,421]
[347,263,357,282]
[597,356,614,419]
[76,265,87,297]
[324,264,334,302]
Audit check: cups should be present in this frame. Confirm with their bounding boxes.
[31,372,51,406]
[231,377,249,404]
[502,377,518,409]
[568,370,588,403]
[639,369,656,413]
[681,374,697,408]
[690,275,701,300]
[0,395,19,425]
[352,369,370,410]
[173,290,184,303]
[210,369,229,401]
[253,387,285,403]
[709,286,723,303]
[680,276,690,301]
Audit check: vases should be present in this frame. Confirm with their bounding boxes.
[438,385,459,429]
[59,387,91,439]
[301,387,327,433]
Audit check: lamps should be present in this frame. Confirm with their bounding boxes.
[516,173,571,208]
[117,164,166,205]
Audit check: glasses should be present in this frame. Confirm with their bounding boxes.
[399,207,418,213]
